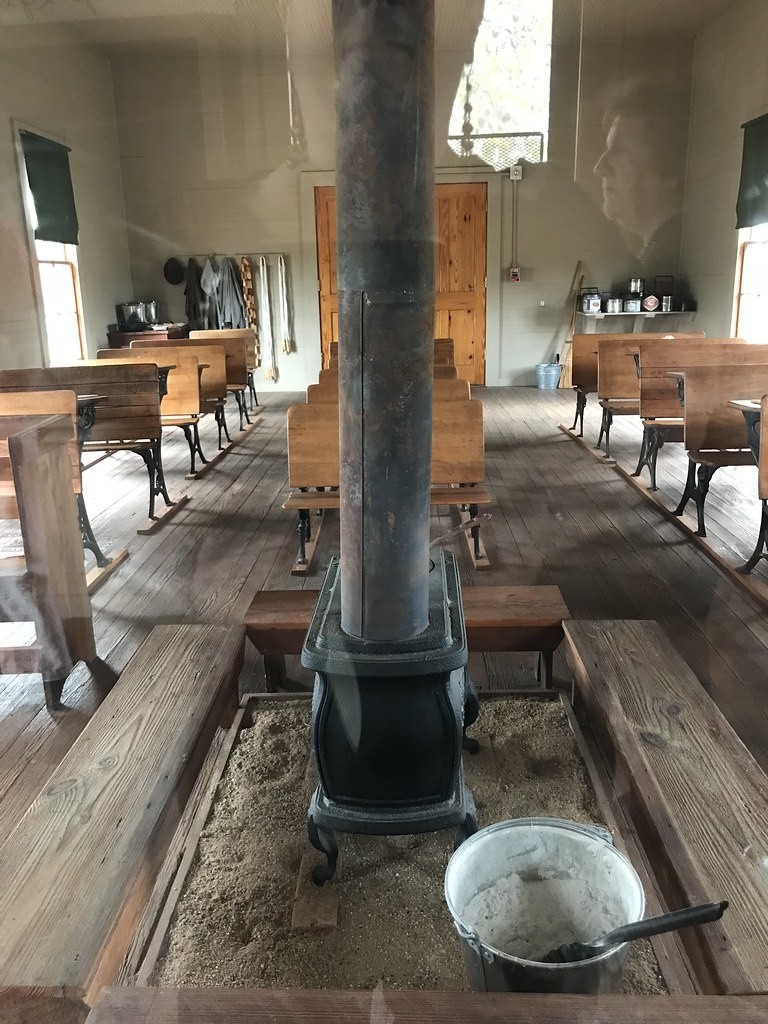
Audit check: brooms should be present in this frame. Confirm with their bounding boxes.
[555,259,583,390]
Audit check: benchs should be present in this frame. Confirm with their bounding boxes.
[561,617,768,994]
[0,622,246,1024]
[82,987,768,1024]
[246,585,569,692]
[0,330,768,577]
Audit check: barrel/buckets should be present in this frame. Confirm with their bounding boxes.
[443,814,646,996]
[606,298,623,312]
[624,299,641,311]
[536,361,563,390]
[121,300,157,324]
[641,290,662,311]
[661,295,674,312]
[627,277,643,294]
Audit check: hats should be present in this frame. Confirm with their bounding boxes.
[163,258,183,284]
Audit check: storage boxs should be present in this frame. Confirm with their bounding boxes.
[143,301,158,324]
[122,301,146,322]
[442,816,645,996]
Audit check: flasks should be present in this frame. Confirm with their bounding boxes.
[583,292,600,312]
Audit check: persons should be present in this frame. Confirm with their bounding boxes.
[238,0,506,339]
[589,81,714,286]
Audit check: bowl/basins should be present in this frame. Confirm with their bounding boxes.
[119,321,147,332]
